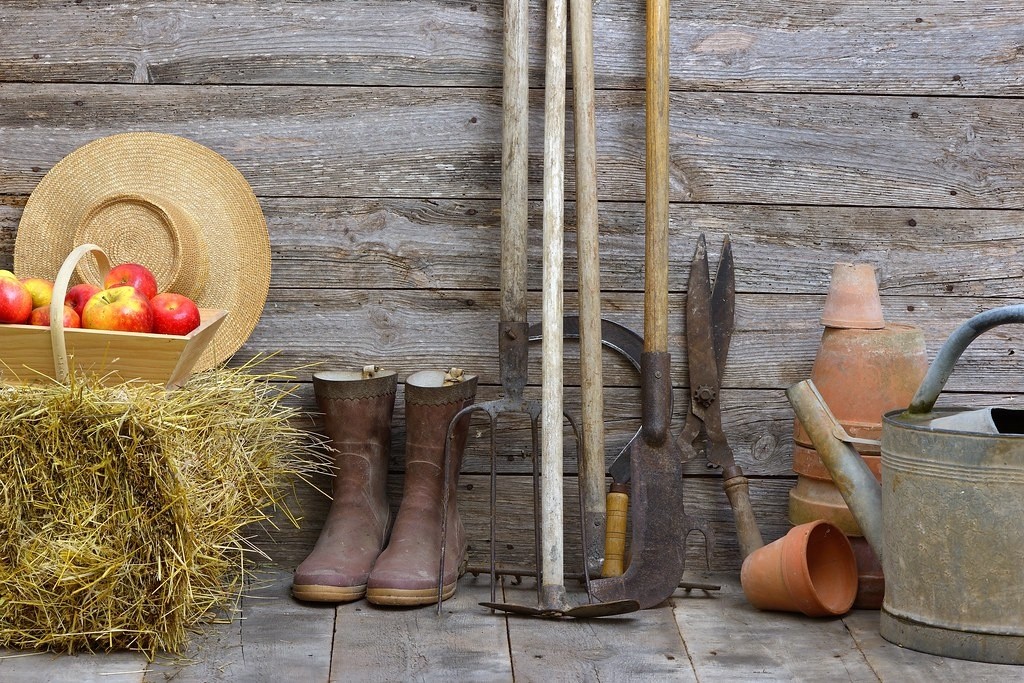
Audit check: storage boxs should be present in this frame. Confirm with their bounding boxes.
[0,243,231,397]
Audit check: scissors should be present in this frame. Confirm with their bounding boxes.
[678,232,766,556]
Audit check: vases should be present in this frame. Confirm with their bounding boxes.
[784,327,931,611]
[740,519,860,619]
[819,262,887,329]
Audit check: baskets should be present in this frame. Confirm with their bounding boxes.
[0,245,229,396]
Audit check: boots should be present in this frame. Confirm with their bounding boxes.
[291,372,479,605]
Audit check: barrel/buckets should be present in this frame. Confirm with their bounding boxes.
[794,325,923,449]
[819,262,884,329]
[741,520,859,611]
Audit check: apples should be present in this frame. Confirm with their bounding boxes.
[0,264,201,336]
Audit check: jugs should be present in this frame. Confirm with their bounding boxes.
[786,306,1024,665]
